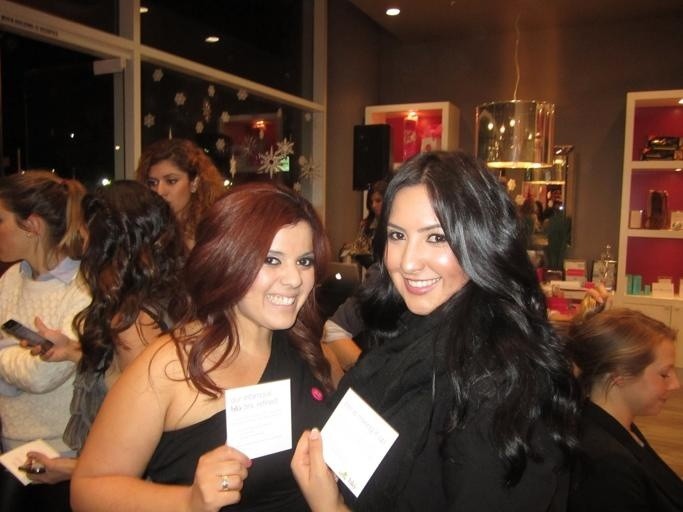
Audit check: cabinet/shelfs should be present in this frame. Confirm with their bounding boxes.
[617,90,683,368]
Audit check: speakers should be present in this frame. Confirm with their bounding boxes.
[353,123,389,191]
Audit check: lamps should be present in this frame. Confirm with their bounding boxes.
[474,13,554,169]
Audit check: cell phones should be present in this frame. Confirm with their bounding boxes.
[0,318,55,354]
[18,461,46,474]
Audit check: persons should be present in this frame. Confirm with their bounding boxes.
[135,136,225,256]
[67,181,343,512]
[0,172,93,510]
[558,305,683,512]
[318,286,412,374]
[293,148,573,512]
[17,178,193,489]
[355,178,389,255]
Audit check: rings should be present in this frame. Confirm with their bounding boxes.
[221,476,231,491]
